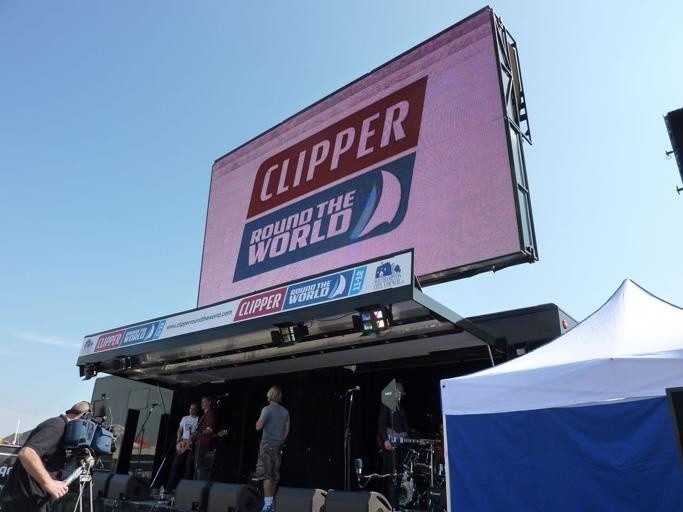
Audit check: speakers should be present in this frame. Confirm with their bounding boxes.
[92,469,112,498]
[175,479,211,512]
[208,483,265,512]
[324,490,392,512]
[109,474,152,500]
[275,487,328,512]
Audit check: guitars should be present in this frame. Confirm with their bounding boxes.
[174,393,229,459]
[379,428,440,449]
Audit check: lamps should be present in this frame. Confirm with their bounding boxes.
[351,303,393,336]
[270,320,309,347]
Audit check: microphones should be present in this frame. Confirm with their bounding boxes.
[216,392,229,398]
[349,385,361,392]
[152,402,162,407]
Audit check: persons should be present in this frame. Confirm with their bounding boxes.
[162,401,199,496]
[189,396,216,480]
[374,382,407,506]
[0,401,94,512]
[254,386,291,512]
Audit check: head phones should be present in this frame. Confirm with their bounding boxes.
[65,409,91,419]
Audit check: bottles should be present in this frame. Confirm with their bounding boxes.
[159,484,164,500]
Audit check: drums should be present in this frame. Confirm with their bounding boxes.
[387,445,446,512]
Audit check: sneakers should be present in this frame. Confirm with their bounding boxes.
[261,504,272,511]
